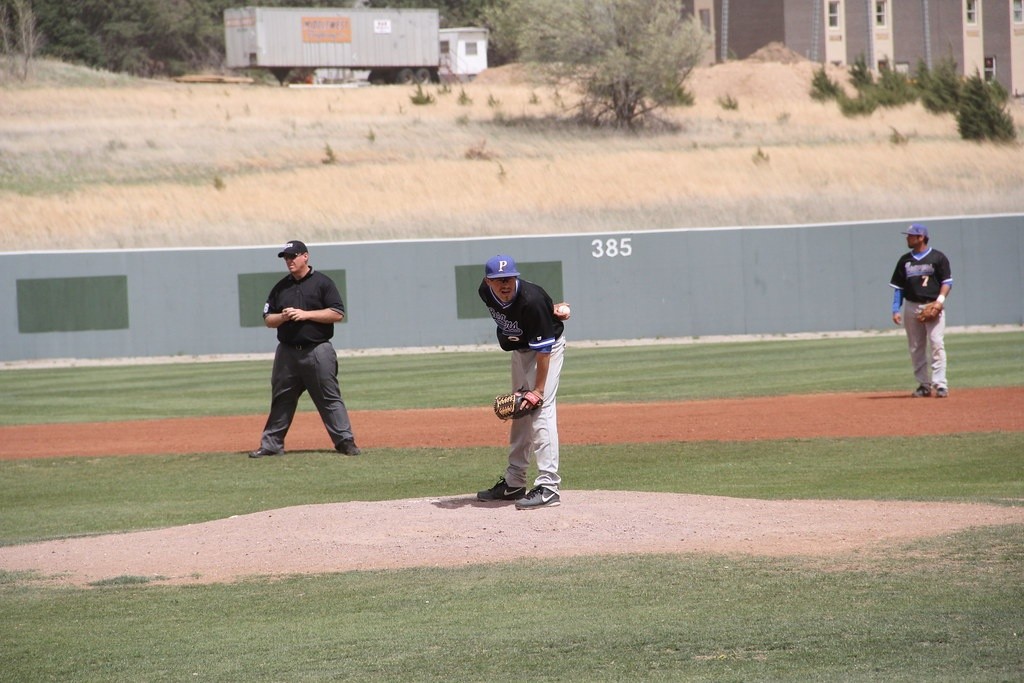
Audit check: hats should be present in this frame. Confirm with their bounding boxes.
[485,255,521,279]
[900,223,928,237]
[278,241,308,258]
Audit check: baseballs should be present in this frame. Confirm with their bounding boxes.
[556,305,571,316]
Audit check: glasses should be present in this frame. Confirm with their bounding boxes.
[487,278,516,283]
[283,252,305,259]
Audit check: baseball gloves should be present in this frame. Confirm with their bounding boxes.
[917,301,942,322]
[494,390,542,420]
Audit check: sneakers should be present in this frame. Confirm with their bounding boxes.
[249,447,284,459]
[936,388,949,398]
[515,485,562,510]
[912,386,932,398]
[477,476,526,501]
[336,439,360,455]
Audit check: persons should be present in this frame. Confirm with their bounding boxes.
[249,241,363,457]
[888,223,954,398]
[476,255,571,509]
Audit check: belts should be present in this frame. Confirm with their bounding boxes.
[281,342,321,350]
